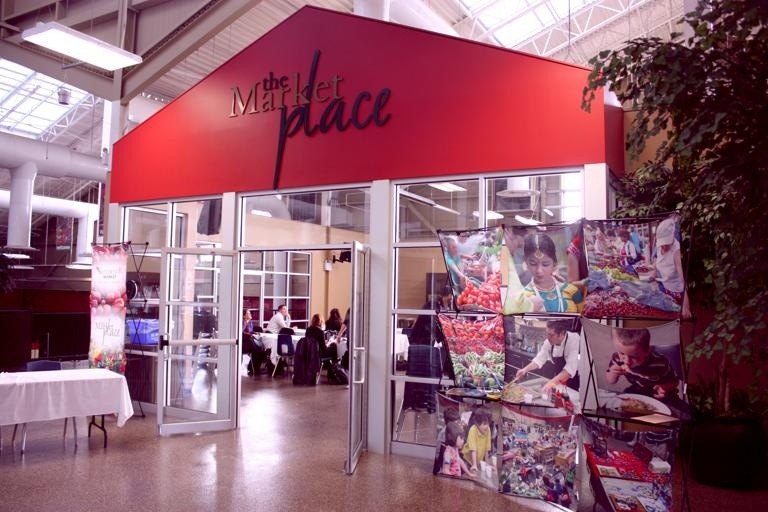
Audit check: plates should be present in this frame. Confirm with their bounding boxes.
[609,392,672,416]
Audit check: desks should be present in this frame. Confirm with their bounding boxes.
[0,368,135,447]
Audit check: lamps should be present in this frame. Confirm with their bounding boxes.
[399,183,437,207]
[58,69,72,105]
[22,1,142,72]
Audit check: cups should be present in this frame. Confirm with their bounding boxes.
[478,455,497,478]
[293,326,299,333]
[523,393,533,404]
[543,392,549,402]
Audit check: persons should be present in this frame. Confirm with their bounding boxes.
[605,326,679,405]
[441,231,501,295]
[242,308,268,376]
[645,432,674,462]
[337,307,350,351]
[516,321,580,394]
[434,401,579,511]
[410,296,444,347]
[503,218,684,313]
[266,304,298,335]
[305,314,338,372]
[325,308,343,332]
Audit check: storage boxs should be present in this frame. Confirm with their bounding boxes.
[127,320,160,345]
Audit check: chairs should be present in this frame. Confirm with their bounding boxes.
[11,360,78,453]
[243,326,349,386]
[394,344,456,442]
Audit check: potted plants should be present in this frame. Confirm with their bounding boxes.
[581,0,768,488]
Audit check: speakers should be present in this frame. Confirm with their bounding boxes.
[341,242,351,262]
[405,345,440,413]
[193,313,217,365]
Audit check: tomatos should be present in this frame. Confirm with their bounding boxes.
[455,272,502,315]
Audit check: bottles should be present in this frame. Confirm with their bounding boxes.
[550,385,573,413]
[30,342,40,359]
